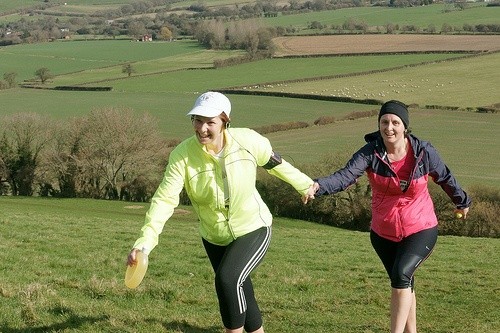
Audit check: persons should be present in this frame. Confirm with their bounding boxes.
[300,99,472,333]
[128,92,316,333]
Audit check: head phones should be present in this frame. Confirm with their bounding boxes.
[225,121,231,129]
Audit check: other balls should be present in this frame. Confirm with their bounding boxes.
[456,212,463,219]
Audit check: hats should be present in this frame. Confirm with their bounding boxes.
[186,91,231,120]
